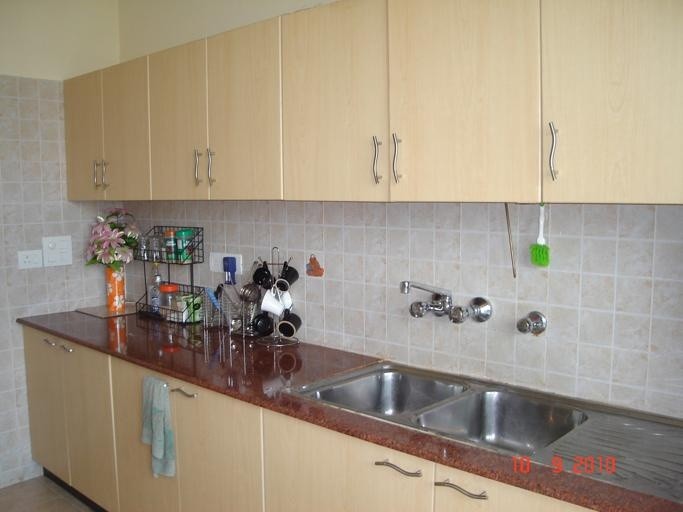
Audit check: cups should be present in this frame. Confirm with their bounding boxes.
[262,291,284,315]
[277,310,301,339]
[253,264,275,289]
[254,311,273,337]
[277,264,299,291]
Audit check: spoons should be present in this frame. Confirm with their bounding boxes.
[240,285,253,336]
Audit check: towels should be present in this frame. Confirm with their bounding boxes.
[139,375,177,479]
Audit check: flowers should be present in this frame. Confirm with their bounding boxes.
[84,207,143,276]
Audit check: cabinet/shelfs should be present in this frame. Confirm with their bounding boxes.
[264,404,603,512]
[544,1,683,206]
[22,323,119,512]
[282,2,542,205]
[60,50,152,206]
[153,12,284,202]
[112,354,263,512]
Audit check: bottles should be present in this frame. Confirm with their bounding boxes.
[159,285,179,318]
[162,231,178,263]
[176,230,193,265]
[150,263,164,314]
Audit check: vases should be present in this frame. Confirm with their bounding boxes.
[106,265,127,317]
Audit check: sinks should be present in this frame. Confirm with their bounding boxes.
[417,389,590,458]
[304,370,468,418]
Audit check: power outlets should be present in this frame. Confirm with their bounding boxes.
[16,248,43,271]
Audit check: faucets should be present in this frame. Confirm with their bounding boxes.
[398,280,454,319]
